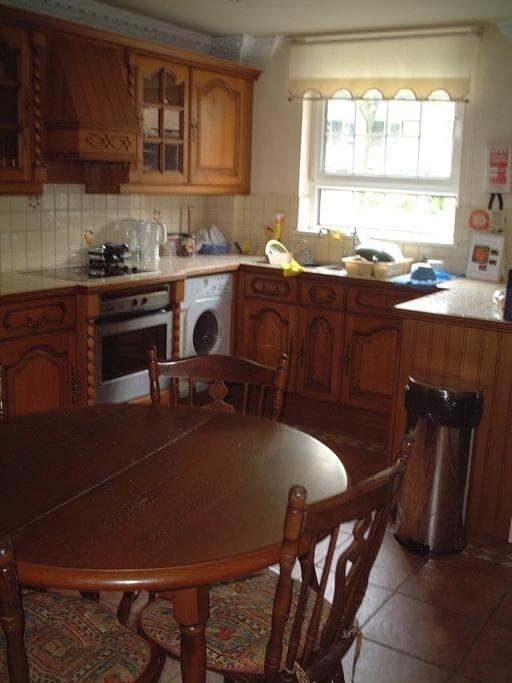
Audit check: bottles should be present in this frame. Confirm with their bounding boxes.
[180,233,195,257]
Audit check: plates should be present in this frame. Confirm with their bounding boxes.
[264,240,290,265]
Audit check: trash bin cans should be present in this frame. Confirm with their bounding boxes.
[395,375,486,554]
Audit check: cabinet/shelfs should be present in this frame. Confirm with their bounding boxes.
[0,286,79,420]
[0,5,43,194]
[85,26,190,194]
[234,271,346,405]
[340,284,428,415]
[190,52,263,195]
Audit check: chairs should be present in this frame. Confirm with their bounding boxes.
[137,436,415,683]
[146,344,290,421]
[0,537,159,683]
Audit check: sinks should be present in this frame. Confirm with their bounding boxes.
[249,256,332,269]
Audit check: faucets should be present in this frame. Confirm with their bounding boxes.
[319,227,347,257]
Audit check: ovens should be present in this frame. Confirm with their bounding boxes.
[96,283,173,403]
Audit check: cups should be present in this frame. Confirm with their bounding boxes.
[161,241,177,275]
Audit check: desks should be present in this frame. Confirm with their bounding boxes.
[0,403,349,626]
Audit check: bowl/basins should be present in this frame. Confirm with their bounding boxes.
[411,266,436,280]
